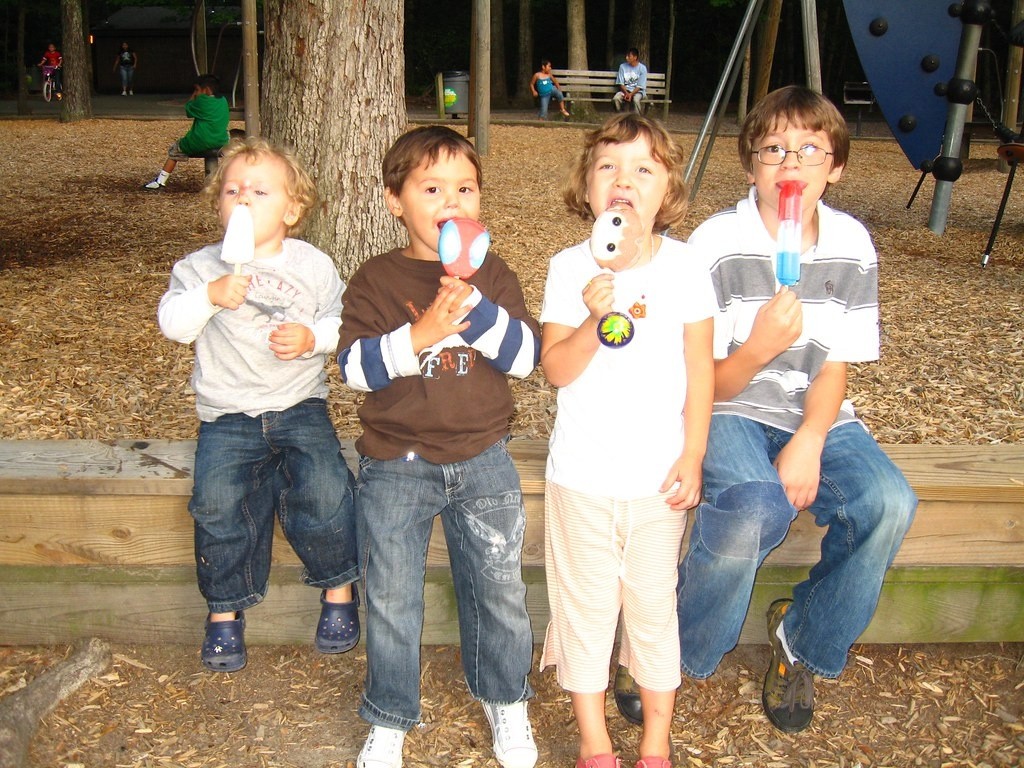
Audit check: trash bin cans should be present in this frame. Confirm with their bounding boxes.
[442,69,470,114]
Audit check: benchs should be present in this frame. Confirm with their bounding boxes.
[169,149,224,180]
[550,69,673,124]
[0,443,1024,656]
[842,75,881,137]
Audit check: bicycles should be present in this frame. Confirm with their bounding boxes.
[38,65,63,103]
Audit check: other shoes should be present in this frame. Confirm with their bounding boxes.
[129,91,133,95]
[122,91,127,95]
[576,754,621,768]
[141,179,166,191]
[560,109,570,116]
[635,756,671,768]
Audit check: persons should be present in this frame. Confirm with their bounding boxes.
[614,84,919,733]
[336,124,543,768]
[530,60,569,123]
[37,43,62,101]
[139,73,230,192]
[113,40,137,96]
[613,49,648,117]
[157,139,358,672]
[539,112,715,768]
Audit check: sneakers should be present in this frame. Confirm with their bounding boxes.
[614,665,644,724]
[483,700,538,768]
[356,725,406,767]
[762,598,814,732]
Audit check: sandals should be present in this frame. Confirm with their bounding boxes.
[201,610,247,671]
[315,582,360,654]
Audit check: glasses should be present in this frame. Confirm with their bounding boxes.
[751,144,834,166]
